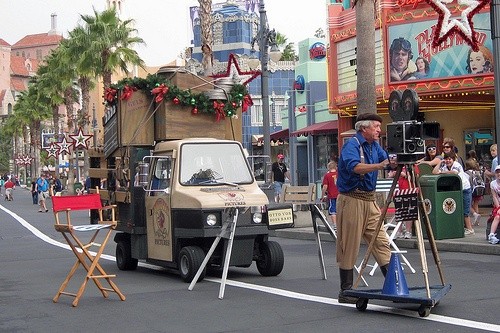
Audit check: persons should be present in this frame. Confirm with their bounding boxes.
[321,161,337,233]
[269,153,290,203]
[387,140,500,244]
[0,173,62,213]
[336,112,398,304]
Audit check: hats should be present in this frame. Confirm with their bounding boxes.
[355,112,383,123]
[391,37,411,50]
[277,154,285,159]
[494,165,500,172]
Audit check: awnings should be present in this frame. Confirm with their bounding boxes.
[290,120,338,137]
[258,128,289,144]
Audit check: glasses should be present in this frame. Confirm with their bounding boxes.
[443,145,451,148]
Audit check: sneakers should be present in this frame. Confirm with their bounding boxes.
[404,232,412,239]
[396,232,403,238]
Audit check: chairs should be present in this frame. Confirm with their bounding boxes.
[50,185,125,307]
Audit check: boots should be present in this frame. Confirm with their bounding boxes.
[338,267,359,304]
[381,264,389,277]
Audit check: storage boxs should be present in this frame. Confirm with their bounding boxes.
[227,102,244,145]
[171,73,214,94]
[108,89,154,158]
[156,99,227,140]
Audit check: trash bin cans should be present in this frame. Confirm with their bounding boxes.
[412,173,464,240]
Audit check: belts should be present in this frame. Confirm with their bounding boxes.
[338,189,377,201]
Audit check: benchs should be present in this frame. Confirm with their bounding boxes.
[281,182,317,210]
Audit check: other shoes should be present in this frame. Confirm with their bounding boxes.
[37,209,43,212]
[46,209,48,212]
[464,228,474,235]
[488,234,499,244]
[472,214,480,226]
[333,224,337,231]
